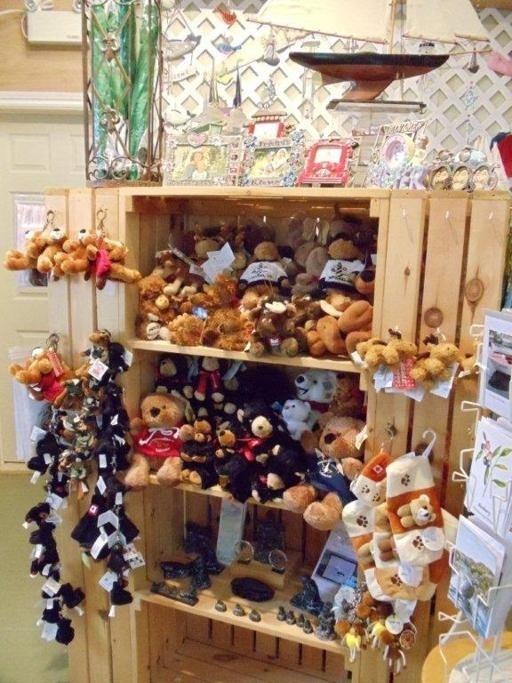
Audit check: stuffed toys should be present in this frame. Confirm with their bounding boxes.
[3,202,465,675]
[2,203,476,678]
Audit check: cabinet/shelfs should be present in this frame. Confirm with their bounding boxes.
[93,187,427,683]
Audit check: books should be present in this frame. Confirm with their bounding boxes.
[447,305,512,641]
